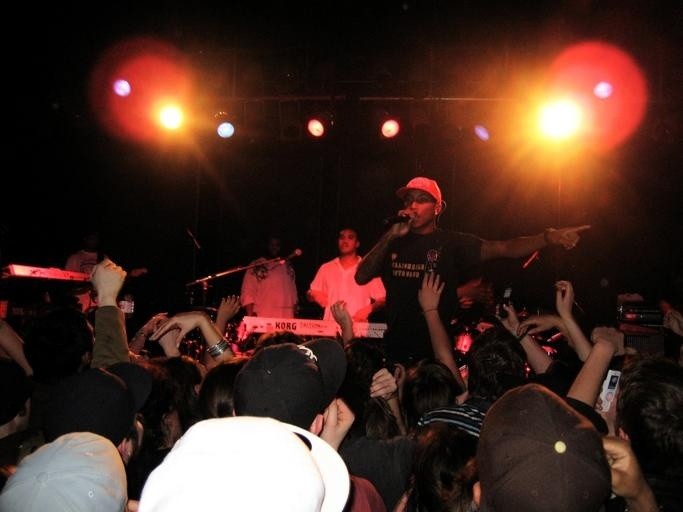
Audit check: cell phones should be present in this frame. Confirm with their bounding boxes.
[499,287,513,318]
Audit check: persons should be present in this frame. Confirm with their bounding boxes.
[242,235,298,319]
[354,176,590,362]
[63,230,108,312]
[307,224,386,322]
[2,260,681,510]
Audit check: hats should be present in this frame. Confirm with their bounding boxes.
[0,432,127,512]
[474,383,611,511]
[138,415,351,512]
[396,177,442,204]
[40,361,152,448]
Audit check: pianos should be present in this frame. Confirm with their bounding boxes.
[240,316,388,340]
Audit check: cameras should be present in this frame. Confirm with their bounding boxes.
[599,370,622,411]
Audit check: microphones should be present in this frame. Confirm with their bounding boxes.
[266,249,302,271]
[382,213,411,228]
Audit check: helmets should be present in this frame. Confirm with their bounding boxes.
[235,340,347,424]
